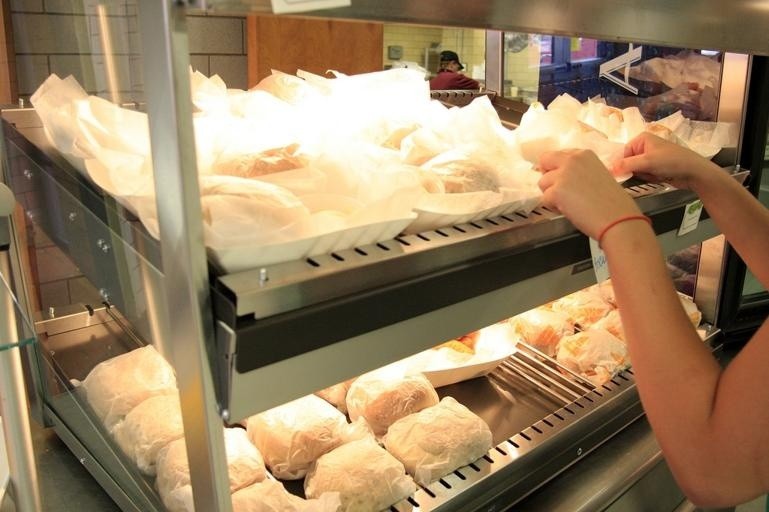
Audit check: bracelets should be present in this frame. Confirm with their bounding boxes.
[597,214,655,248]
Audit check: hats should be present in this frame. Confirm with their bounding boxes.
[440,51,464,70]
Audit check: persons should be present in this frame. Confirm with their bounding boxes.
[538,130,768,512]
[430,50,481,89]
